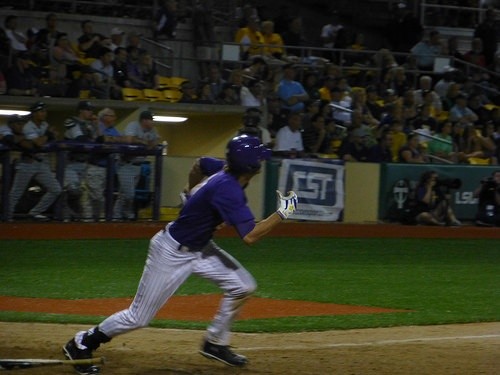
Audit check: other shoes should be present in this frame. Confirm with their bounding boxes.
[30,213,47,219]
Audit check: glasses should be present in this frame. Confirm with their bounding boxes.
[105,115,116,118]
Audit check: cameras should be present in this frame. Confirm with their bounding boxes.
[431,177,461,191]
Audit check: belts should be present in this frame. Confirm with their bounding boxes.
[123,158,141,166]
[163,221,201,253]
[90,162,108,168]
[70,158,85,163]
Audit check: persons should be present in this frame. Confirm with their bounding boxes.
[63,134,299,375]
[0,0,500,227]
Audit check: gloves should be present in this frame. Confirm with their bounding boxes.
[180,190,190,205]
[276,190,298,220]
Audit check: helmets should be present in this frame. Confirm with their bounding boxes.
[226,134,272,165]
[30,101,47,112]
[7,115,25,124]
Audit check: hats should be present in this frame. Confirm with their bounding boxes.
[80,101,96,109]
[128,31,143,39]
[352,129,370,136]
[139,111,154,119]
[111,27,124,35]
[367,84,378,92]
[282,62,300,70]
[322,76,338,81]
[27,27,40,39]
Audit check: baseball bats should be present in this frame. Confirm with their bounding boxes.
[0,356,108,369]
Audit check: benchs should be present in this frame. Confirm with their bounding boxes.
[0,33,500,203]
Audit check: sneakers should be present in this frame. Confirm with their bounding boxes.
[198,340,248,367]
[62,337,101,375]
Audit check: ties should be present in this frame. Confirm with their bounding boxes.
[13,31,27,43]
